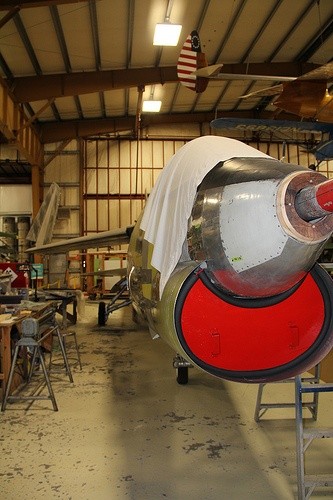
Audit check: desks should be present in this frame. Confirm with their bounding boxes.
[0,300,49,394]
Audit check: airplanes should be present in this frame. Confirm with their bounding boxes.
[23,133,333,385]
[189,58,333,124]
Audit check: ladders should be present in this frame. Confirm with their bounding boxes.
[26,180,62,247]
